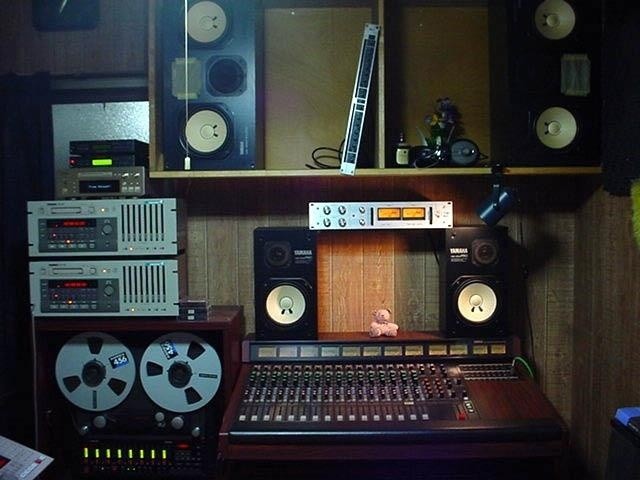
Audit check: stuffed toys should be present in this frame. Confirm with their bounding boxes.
[369,307,399,338]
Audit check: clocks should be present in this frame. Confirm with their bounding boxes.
[31,0,101,32]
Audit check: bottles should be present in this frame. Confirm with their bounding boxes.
[392,131,415,169]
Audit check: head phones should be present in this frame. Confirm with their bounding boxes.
[410,139,480,165]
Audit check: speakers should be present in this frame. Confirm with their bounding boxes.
[490,4,598,165]
[160,1,262,167]
[438,226,512,342]
[253,226,318,343]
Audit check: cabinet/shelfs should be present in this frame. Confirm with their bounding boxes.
[147,0,605,180]
